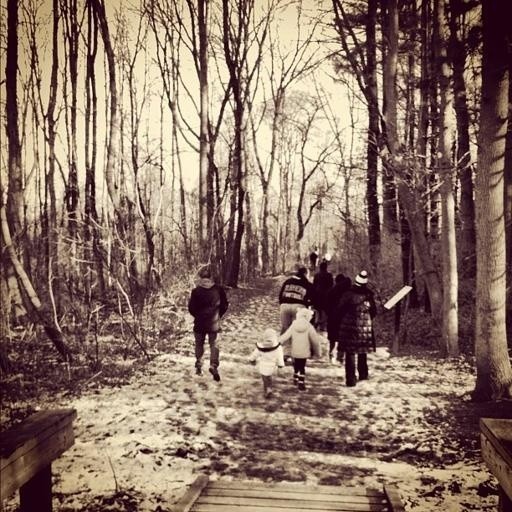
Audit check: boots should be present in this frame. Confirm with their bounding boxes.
[293,373,306,391]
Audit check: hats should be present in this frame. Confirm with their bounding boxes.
[353,270,368,286]
[263,328,277,342]
[296,307,314,321]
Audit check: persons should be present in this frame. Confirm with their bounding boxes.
[325,273,347,359]
[277,266,316,335]
[247,328,286,399]
[333,276,353,308]
[314,247,320,270]
[319,257,328,273]
[310,262,334,331]
[330,268,378,386]
[309,252,318,269]
[188,270,229,381]
[277,306,322,391]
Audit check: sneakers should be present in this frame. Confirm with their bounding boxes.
[265,388,272,398]
[329,352,344,363]
[196,365,220,381]
[346,376,369,387]
